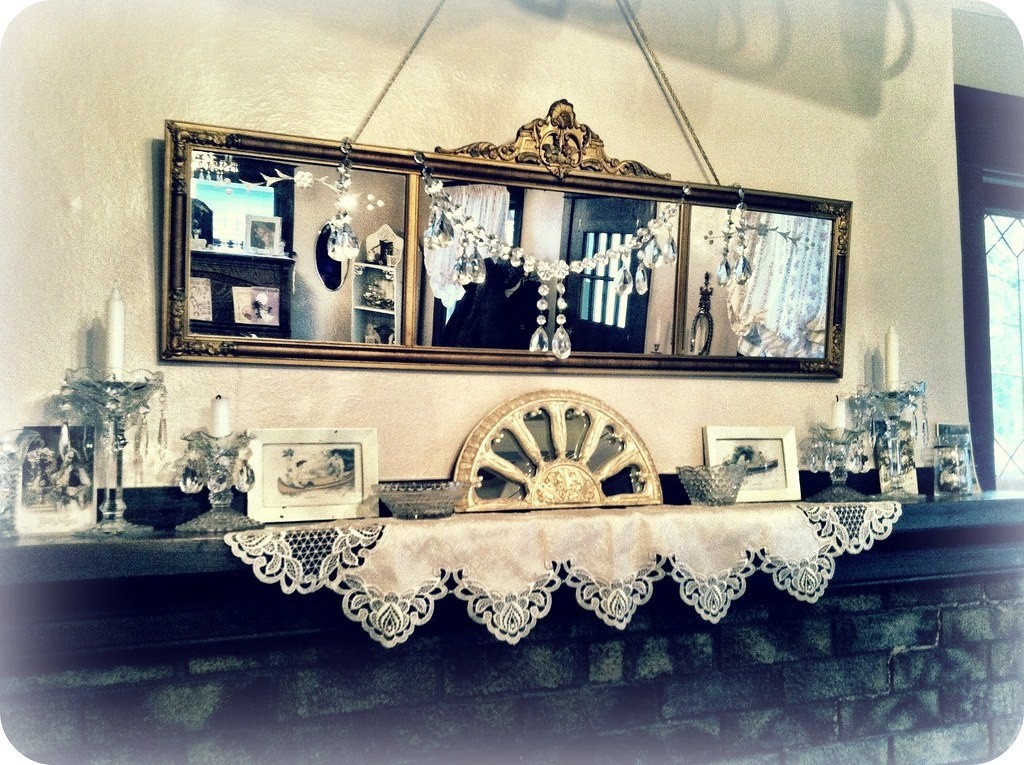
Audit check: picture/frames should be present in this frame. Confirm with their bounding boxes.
[702,424,807,508]
[235,425,385,524]
[154,117,850,388]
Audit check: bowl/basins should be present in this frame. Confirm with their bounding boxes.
[676,462,749,507]
[369,479,472,521]
